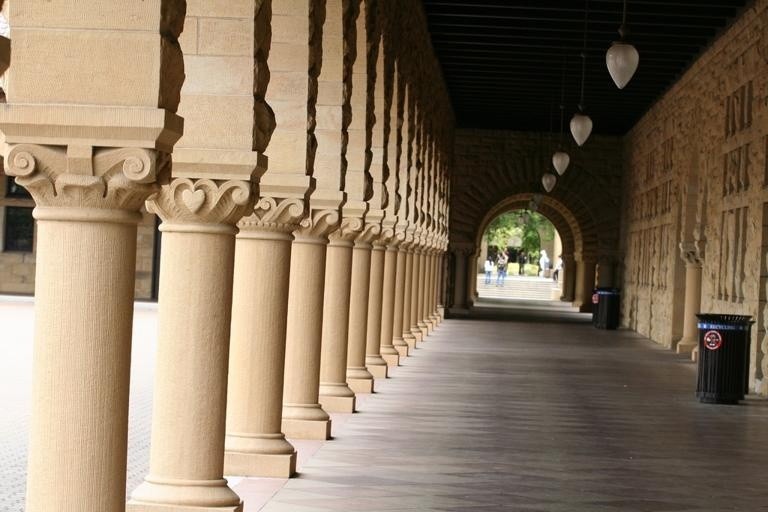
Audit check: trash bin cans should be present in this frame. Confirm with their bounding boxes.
[592,286,621,330]
[696,313,756,405]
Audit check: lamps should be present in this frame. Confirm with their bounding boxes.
[523,206,529,224]
[569,0,593,148]
[517,207,523,226]
[606,0,639,91]
[528,194,537,213]
[541,148,556,193]
[552,48,570,177]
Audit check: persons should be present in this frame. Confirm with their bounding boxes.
[554,255,563,281]
[484,248,509,287]
[518,252,527,275]
